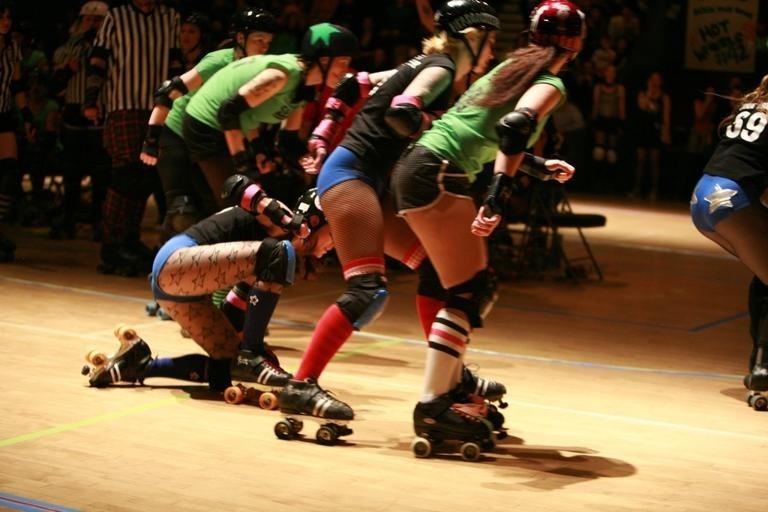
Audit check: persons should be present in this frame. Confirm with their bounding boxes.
[691,75,768,411]
[1,0,222,278]
[530,1,752,204]
[81,2,587,459]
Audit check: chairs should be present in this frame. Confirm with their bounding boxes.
[519,186,607,280]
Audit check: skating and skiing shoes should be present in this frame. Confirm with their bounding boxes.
[83,324,151,388]
[458,364,507,409]
[450,390,507,443]
[269,376,356,445]
[412,391,490,462]
[743,367,766,407]
[98,236,155,276]
[224,346,292,409]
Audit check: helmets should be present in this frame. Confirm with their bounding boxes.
[433,0,503,34]
[80,1,108,17]
[302,19,355,65]
[530,2,589,53]
[295,185,329,229]
[180,11,206,31]
[231,7,275,34]
